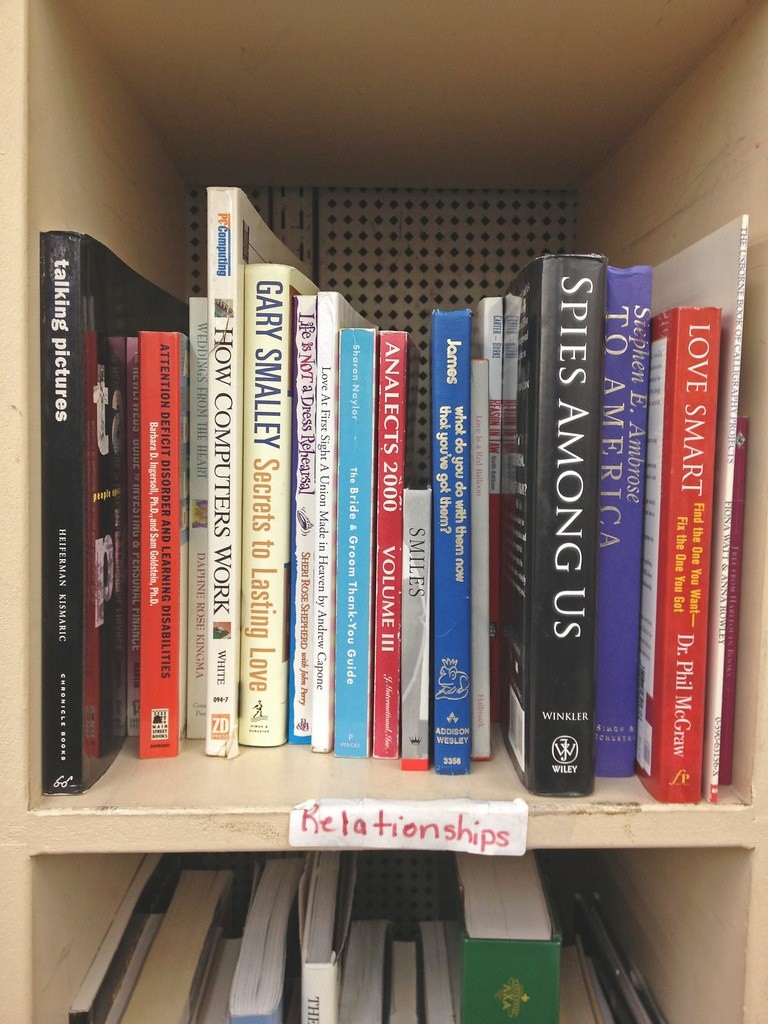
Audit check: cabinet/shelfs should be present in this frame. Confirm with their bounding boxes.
[0,0,768,1024]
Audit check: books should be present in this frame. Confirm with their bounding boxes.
[40,184,750,807]
[68,853,672,1023]
